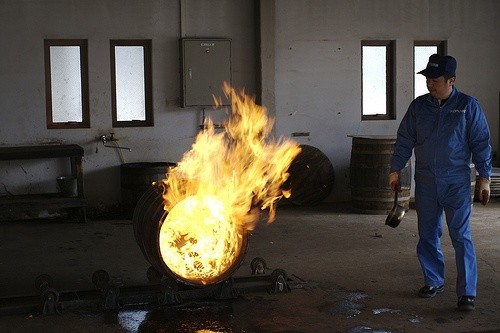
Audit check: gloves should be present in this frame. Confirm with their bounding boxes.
[388,172,403,193]
[476,177,491,204]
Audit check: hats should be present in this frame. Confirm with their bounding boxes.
[417,54,457,78]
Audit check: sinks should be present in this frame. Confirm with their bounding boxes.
[121,162,176,177]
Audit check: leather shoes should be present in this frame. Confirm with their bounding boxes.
[418,284,444,297]
[457,295,475,311]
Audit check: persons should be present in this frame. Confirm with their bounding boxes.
[389,53,492,312]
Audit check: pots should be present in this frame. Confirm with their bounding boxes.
[384,184,407,228]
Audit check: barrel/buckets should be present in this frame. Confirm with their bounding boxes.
[131,185,249,288]
[349,135,411,215]
[57,175,80,197]
[120,162,177,212]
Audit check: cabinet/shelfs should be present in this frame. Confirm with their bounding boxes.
[0,144,87,225]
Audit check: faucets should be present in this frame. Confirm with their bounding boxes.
[100,132,131,152]
